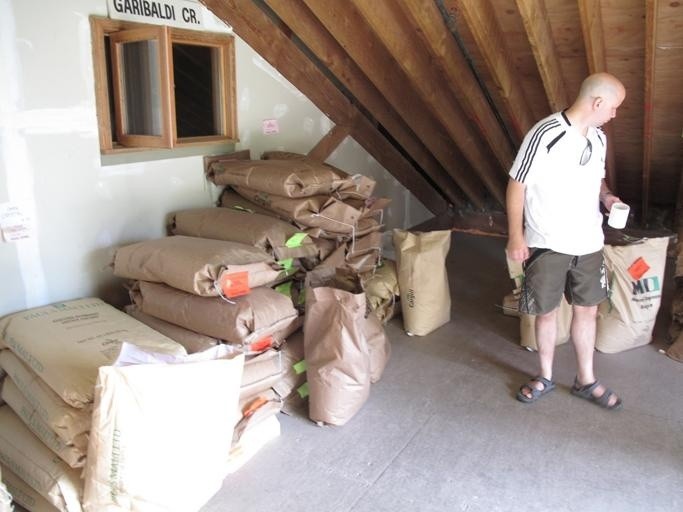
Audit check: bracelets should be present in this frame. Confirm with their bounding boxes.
[602,192,614,204]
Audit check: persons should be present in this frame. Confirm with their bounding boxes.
[506,72,626,411]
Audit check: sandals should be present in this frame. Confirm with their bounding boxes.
[516,376,556,403]
[572,375,624,410]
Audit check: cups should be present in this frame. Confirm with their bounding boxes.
[607,202,630,230]
